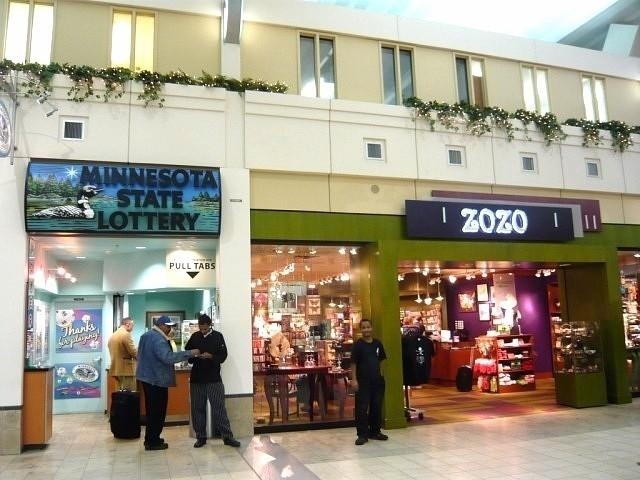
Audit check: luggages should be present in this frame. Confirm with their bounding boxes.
[109,356,141,440]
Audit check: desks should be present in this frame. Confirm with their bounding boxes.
[307,368,349,419]
[252,361,332,425]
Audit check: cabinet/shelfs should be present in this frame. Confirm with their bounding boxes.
[620,274,640,352]
[19,362,54,449]
[485,332,537,395]
[551,320,608,407]
[253,308,442,368]
[103,364,195,424]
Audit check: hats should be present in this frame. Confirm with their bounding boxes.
[156,316,176,326]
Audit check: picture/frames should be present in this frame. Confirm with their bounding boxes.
[474,281,491,303]
[477,303,492,323]
[144,309,186,345]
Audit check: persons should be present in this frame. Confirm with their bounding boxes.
[348,319,393,446]
[399,314,435,390]
[184,312,241,449]
[106,316,138,394]
[134,315,201,449]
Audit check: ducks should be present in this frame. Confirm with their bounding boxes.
[33,184,103,218]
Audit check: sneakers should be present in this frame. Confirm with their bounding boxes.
[356,436,367,445]
[370,432,388,440]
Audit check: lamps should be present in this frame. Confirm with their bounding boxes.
[253,245,360,293]
[0,73,60,119]
[397,262,496,307]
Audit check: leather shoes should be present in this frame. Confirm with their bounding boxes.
[194,439,206,448]
[224,440,240,447]
[144,438,168,449]
